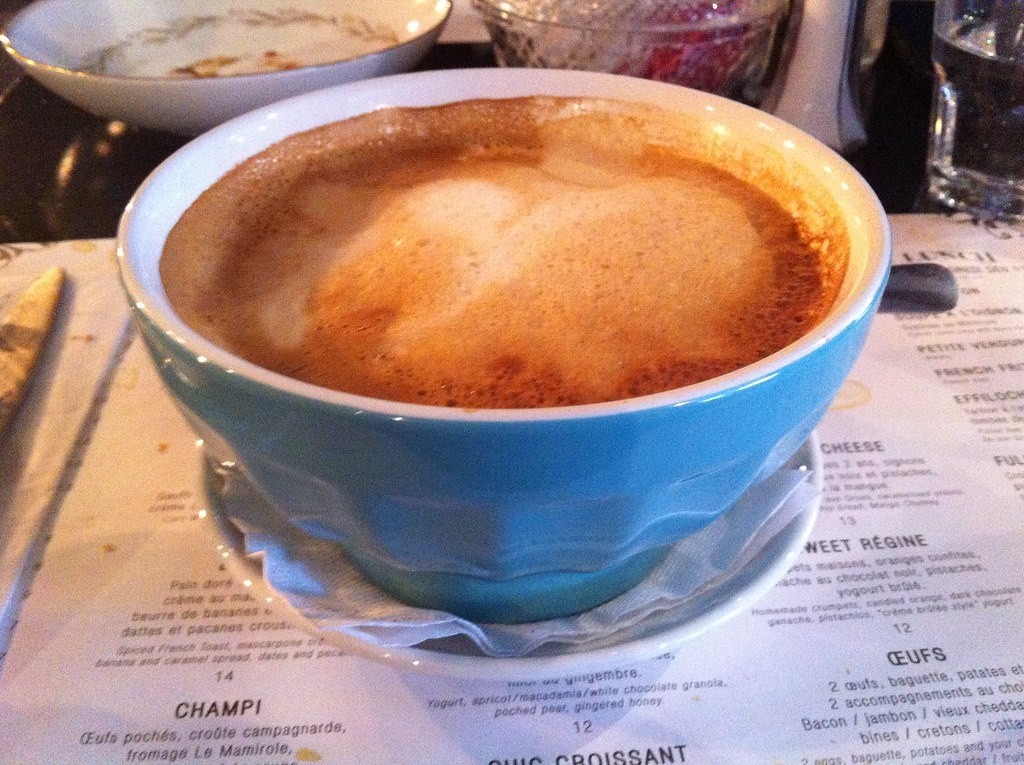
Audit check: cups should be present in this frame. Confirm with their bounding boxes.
[470,0,804,120]
[925,0,1024,220]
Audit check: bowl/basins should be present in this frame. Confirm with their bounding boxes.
[0,0,453,138]
[116,68,896,624]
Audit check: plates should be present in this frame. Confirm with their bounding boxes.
[0,41,524,243]
[192,429,824,684]
